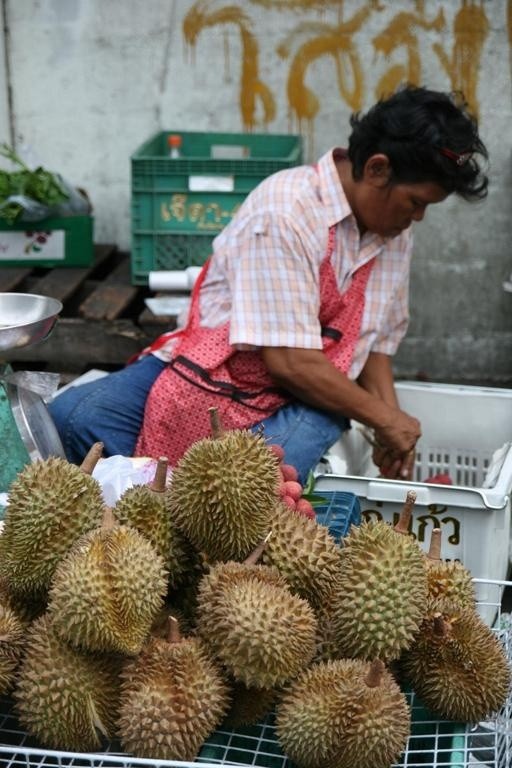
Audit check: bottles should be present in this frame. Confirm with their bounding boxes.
[167,136,185,157]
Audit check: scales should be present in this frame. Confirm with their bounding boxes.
[0,292,69,521]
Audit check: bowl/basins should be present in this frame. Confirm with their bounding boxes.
[0,291,69,352]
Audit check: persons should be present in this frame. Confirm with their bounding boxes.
[45,82,491,491]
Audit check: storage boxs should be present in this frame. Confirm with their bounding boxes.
[0,194,97,271]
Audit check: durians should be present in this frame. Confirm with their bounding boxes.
[0,428,512,768]
